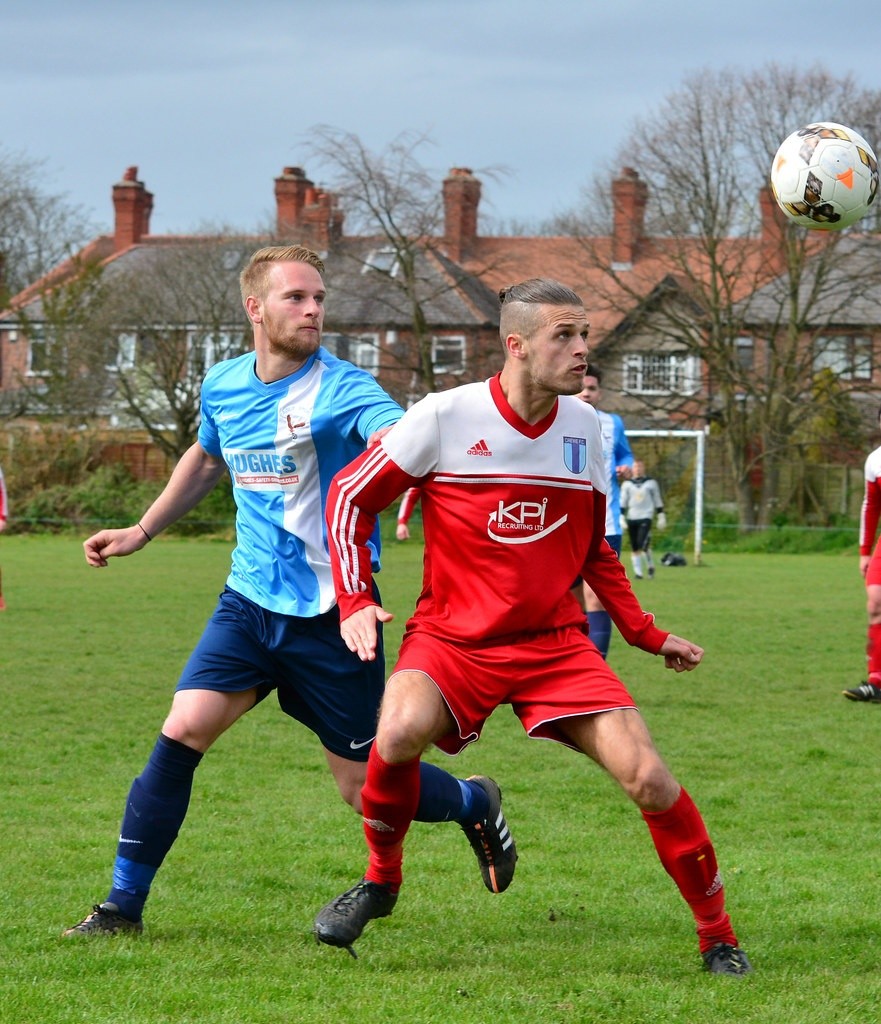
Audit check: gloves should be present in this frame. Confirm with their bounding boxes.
[619,515,628,530]
[656,513,667,528]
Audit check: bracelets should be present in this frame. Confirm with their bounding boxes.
[137,523,153,542]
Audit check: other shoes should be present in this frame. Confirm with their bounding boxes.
[646,567,655,578]
[633,574,643,580]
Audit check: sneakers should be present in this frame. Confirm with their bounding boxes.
[463,775,516,890]
[64,908,141,941]
[844,680,880,703]
[316,879,397,945]
[703,945,750,978]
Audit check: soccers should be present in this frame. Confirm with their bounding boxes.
[766,121,878,230]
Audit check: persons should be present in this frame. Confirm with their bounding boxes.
[56,245,521,946]
[308,274,751,980]
[841,405,881,706]
[573,360,638,661]
[619,458,667,580]
